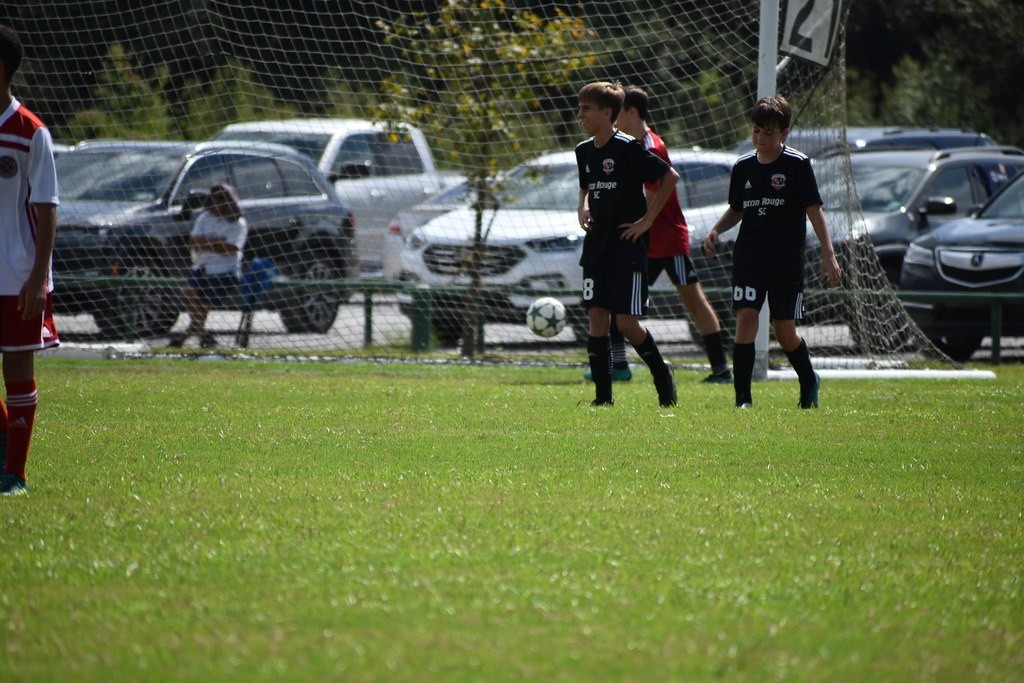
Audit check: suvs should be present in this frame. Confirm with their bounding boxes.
[50,138,357,343]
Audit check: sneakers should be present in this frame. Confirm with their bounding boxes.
[798,372,820,410]
[702,367,733,383]
[0,474,26,495]
[736,404,752,410]
[652,360,678,407]
[182,335,203,350]
[583,367,632,381]
[170,312,191,333]
[577,399,615,412]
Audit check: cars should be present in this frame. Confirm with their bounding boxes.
[382,171,497,295]
[396,125,1024,363]
[202,117,469,296]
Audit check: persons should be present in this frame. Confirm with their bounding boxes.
[0,26,59,495]
[168,186,249,349]
[705,94,842,410]
[584,84,732,384]
[574,80,679,409]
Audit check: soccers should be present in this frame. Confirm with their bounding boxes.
[526,296,566,338]
